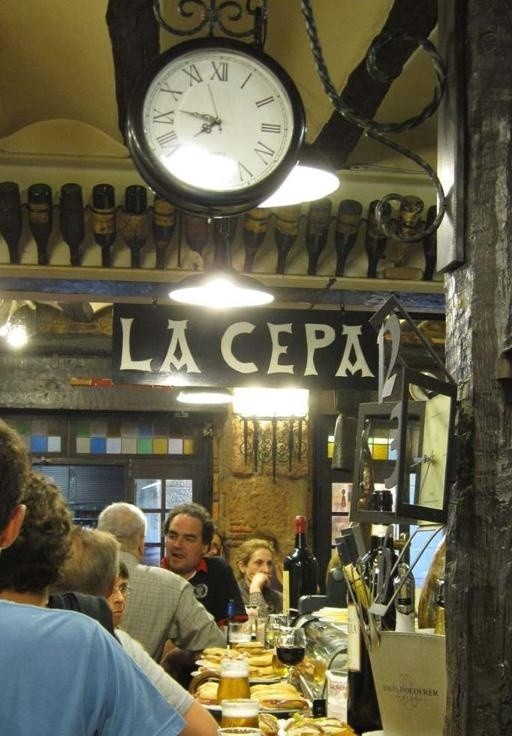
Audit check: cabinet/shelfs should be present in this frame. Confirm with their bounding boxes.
[0,151,449,314]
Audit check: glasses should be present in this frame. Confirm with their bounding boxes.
[112,585,132,594]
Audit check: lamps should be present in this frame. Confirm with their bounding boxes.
[175,387,234,405]
[232,384,310,483]
[258,144,340,209]
[166,265,274,308]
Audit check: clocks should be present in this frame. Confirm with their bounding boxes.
[104,0,307,218]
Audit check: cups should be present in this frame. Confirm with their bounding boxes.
[229,622,252,648]
[217,668,251,703]
[276,628,307,683]
[221,699,259,728]
[217,727,262,736]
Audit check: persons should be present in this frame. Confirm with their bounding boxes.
[0,472,122,645]
[246,530,283,592]
[208,525,224,557]
[159,505,247,689]
[51,525,119,599]
[231,539,282,618]
[97,502,227,667]
[0,418,187,735]
[105,560,220,736]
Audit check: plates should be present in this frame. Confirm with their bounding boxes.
[196,696,312,714]
[196,659,206,666]
[190,671,288,682]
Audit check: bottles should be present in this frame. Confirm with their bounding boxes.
[347,489,399,733]
[222,599,238,645]
[282,516,319,616]
